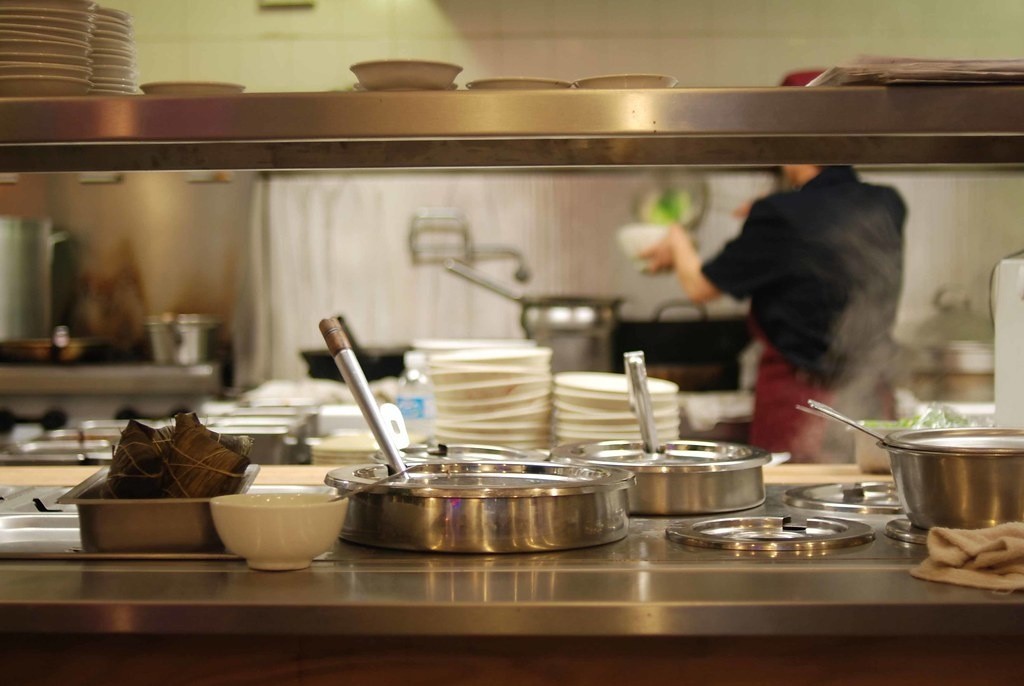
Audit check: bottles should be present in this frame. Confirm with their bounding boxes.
[395,350,435,447]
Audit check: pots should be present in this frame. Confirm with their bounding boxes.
[0,326,106,364]
[612,298,757,366]
[143,312,222,365]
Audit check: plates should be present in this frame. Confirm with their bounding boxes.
[139,81,247,94]
[402,332,682,453]
[574,74,679,90]
[465,78,573,90]
[0,0,138,97]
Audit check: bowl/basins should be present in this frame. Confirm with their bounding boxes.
[349,60,463,90]
[208,492,349,572]
[614,223,701,277]
[877,441,1024,531]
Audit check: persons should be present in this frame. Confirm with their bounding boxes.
[636,68,908,466]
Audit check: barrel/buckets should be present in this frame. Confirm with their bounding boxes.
[521,297,623,374]
